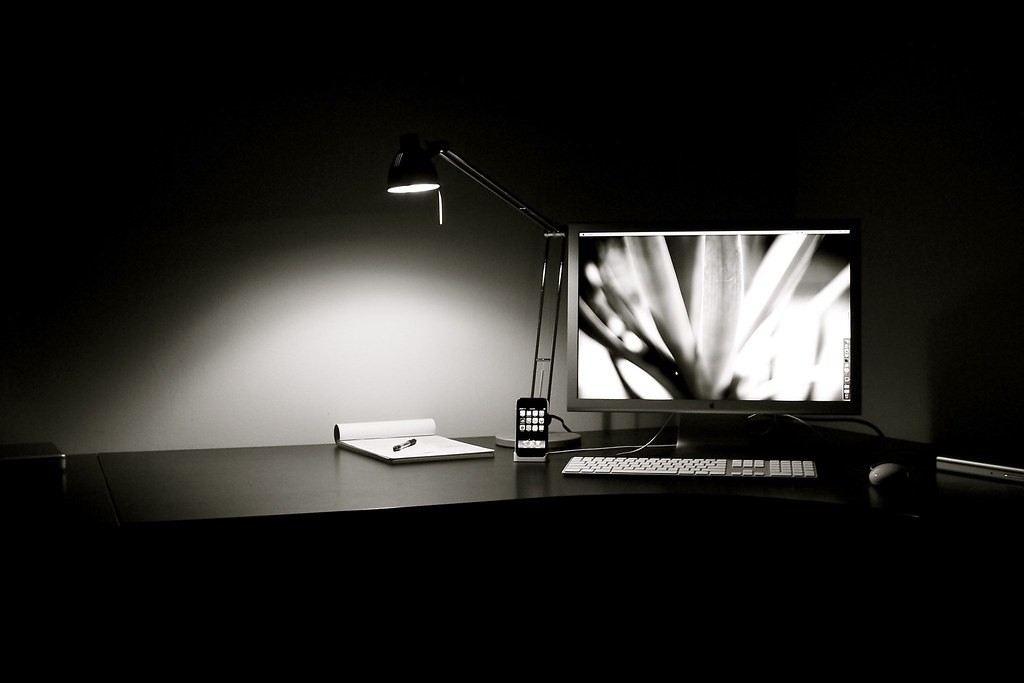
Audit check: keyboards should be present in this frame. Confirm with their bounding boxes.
[561,455,818,479]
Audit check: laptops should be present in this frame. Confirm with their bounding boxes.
[934,453,1024,483]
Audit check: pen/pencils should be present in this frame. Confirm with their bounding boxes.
[393,438,417,452]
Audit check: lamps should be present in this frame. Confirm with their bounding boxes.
[386,130,581,450]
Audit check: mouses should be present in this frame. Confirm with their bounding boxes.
[869,463,908,485]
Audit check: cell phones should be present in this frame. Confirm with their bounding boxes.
[515,398,549,457]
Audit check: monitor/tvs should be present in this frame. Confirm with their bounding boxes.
[566,215,870,455]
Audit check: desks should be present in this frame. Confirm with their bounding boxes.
[97,426,1024,683]
[0,454,121,683]
